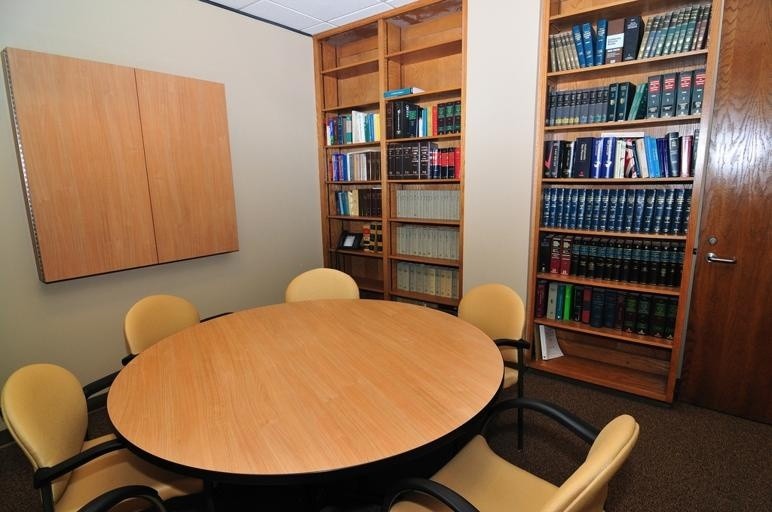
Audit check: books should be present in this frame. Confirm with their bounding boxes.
[324,87,461,309]
[546,69,706,126]
[549,4,712,74]
[539,234,685,287]
[543,128,699,179]
[535,280,678,340]
[542,188,692,235]
[538,325,564,360]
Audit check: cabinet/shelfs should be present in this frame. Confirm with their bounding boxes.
[520,0,724,403]
[311,0,468,310]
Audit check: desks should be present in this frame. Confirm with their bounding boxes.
[108,298,507,481]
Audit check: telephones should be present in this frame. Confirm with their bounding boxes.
[337,231,363,249]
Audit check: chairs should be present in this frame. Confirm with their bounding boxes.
[373,399,640,511]
[119,295,234,370]
[0,362,205,512]
[287,268,359,303]
[455,283,530,450]
